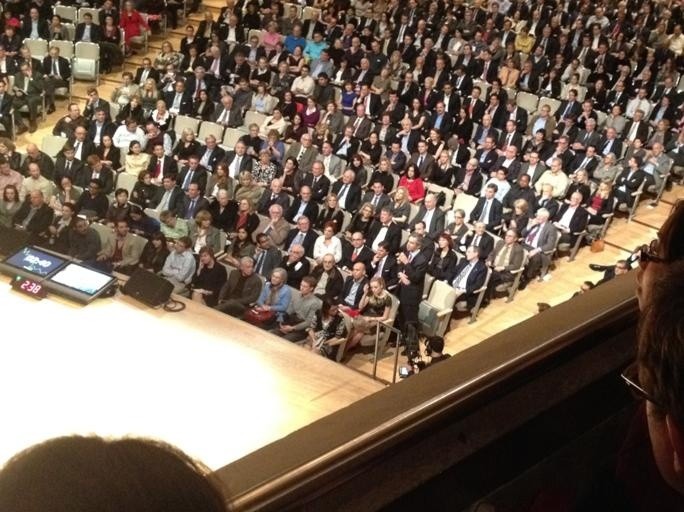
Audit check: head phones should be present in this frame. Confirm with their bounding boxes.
[423,338,433,357]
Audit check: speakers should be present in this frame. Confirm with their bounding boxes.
[122,266,176,308]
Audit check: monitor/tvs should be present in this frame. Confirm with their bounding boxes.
[40,261,119,304]
[0,244,73,283]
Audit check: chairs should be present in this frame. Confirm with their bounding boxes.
[0,0,682,383]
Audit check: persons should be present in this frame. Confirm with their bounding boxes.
[419,336,452,369]
[538,303,550,312]
[195,0,684,256]
[0,0,205,139]
[619,266,684,494]
[633,197,683,314]
[573,281,594,299]
[0,126,557,360]
[0,432,235,511]
[615,260,628,275]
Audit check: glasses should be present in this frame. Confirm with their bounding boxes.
[618,361,684,417]
[637,234,671,267]
